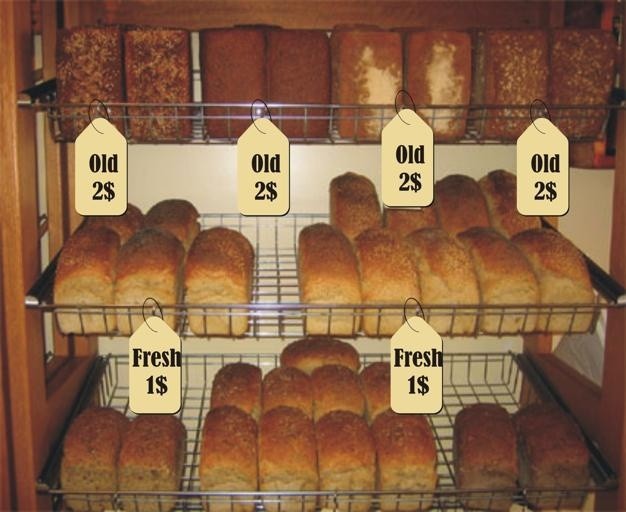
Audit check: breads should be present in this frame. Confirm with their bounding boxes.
[457,225,538,333]
[198,406,257,512]
[256,408,317,511]
[405,33,472,141]
[452,402,517,511]
[142,200,200,267]
[54,25,123,143]
[82,204,143,243]
[407,228,479,334]
[261,367,314,418]
[265,29,328,144]
[329,174,381,241]
[370,407,437,511]
[116,230,187,334]
[330,30,404,143]
[550,29,614,141]
[54,228,120,334]
[208,364,261,419]
[199,29,263,140]
[279,337,358,374]
[384,206,437,239]
[315,411,376,510]
[511,230,595,334]
[310,364,366,422]
[298,224,363,336]
[60,407,129,511]
[360,361,391,421]
[119,414,189,511]
[513,403,588,511]
[474,31,546,142]
[479,171,542,241]
[189,228,252,338]
[123,28,192,143]
[432,175,490,238]
[353,230,420,336]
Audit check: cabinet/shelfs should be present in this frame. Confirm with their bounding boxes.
[0,0,626,512]
